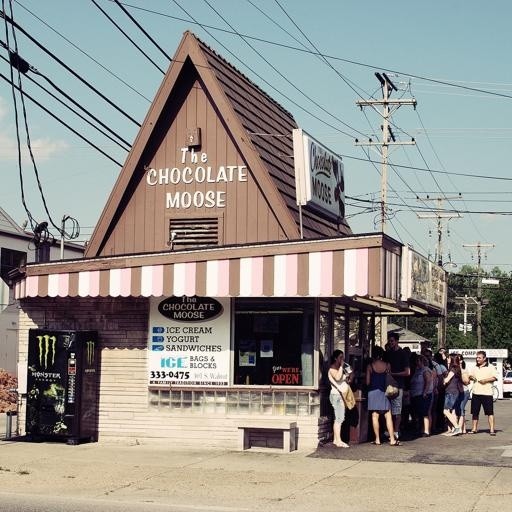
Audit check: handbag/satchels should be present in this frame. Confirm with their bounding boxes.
[385,370,399,397]
[341,387,356,410]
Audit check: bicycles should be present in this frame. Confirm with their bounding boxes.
[468,380,500,403]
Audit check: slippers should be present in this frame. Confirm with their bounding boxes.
[390,440,403,446]
[468,429,478,434]
[489,430,496,436]
[370,441,380,445]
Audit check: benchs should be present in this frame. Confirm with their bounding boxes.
[237,421,297,452]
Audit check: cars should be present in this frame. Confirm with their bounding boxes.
[501,371,512,400]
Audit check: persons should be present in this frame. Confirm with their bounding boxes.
[364,331,471,446]
[465,349,500,437]
[325,349,357,449]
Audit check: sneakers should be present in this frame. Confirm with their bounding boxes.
[442,427,467,436]
[333,441,349,448]
